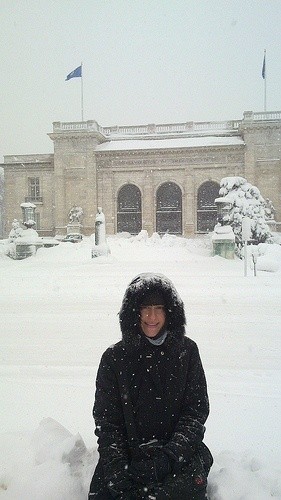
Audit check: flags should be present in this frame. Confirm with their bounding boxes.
[262,55,265,79]
[64,65,81,81]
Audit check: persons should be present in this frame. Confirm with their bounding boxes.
[86,272,215,500]
[94,206,107,244]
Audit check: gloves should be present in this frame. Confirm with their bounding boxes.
[102,456,170,500]
